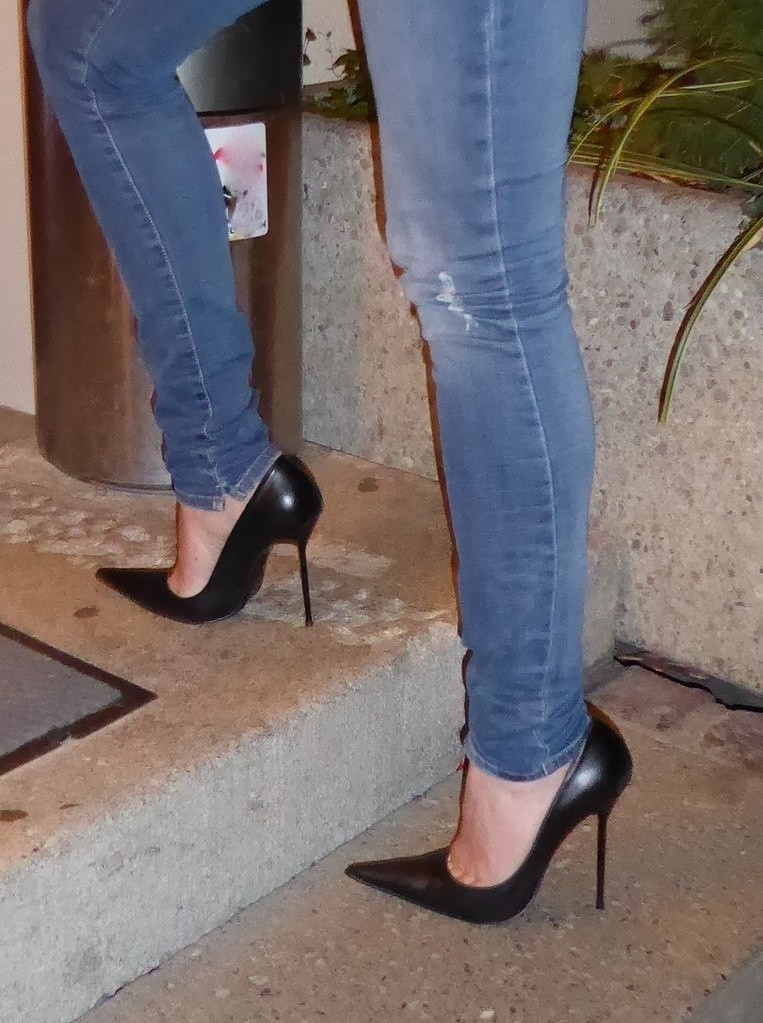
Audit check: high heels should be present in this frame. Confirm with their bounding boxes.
[94,453,325,627]
[344,714,633,926]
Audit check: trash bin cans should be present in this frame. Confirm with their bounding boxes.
[23,0,304,491]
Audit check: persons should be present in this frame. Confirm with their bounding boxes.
[29,1,634,923]
